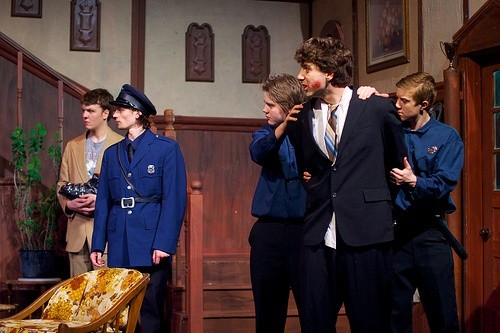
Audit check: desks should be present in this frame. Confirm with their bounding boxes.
[0,279,62,317]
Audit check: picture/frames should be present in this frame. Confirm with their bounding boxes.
[364,0,409,73]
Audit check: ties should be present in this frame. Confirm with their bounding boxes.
[127,143,134,164]
[324,105,337,162]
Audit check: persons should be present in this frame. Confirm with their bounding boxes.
[248,73,311,333]
[287,36,409,333]
[56,84,187,333]
[357,72,467,333]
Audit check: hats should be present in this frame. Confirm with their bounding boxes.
[108,83,157,118]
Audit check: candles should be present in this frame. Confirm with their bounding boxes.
[57,81,64,118]
[18,52,22,87]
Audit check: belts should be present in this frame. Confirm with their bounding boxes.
[112,197,153,208]
[260,217,303,225]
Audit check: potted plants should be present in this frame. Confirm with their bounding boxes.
[9,121,66,278]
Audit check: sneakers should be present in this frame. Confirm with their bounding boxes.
[58,173,99,201]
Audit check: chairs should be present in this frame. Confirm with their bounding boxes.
[0,268,151,333]
